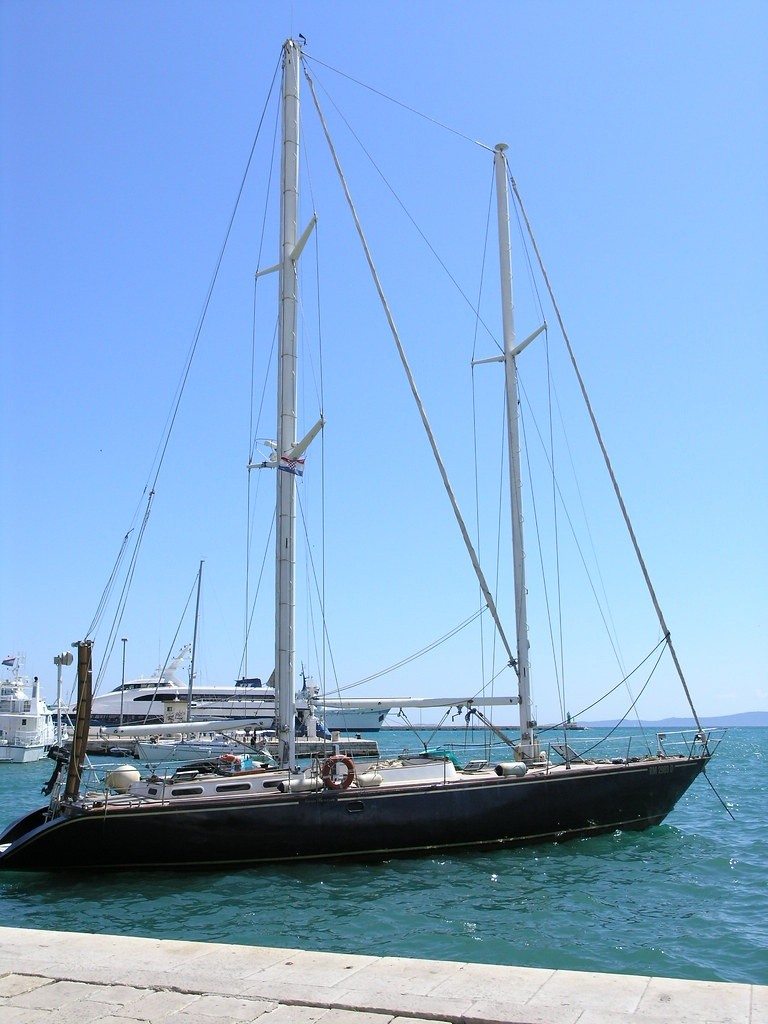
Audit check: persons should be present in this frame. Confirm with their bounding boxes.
[250,733,267,749]
[149,735,156,744]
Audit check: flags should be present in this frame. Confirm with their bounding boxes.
[1,656,16,666]
[277,455,305,477]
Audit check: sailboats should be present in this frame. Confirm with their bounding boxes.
[0,34,720,879]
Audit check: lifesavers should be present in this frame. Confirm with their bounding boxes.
[322,755,355,790]
[220,754,242,771]
